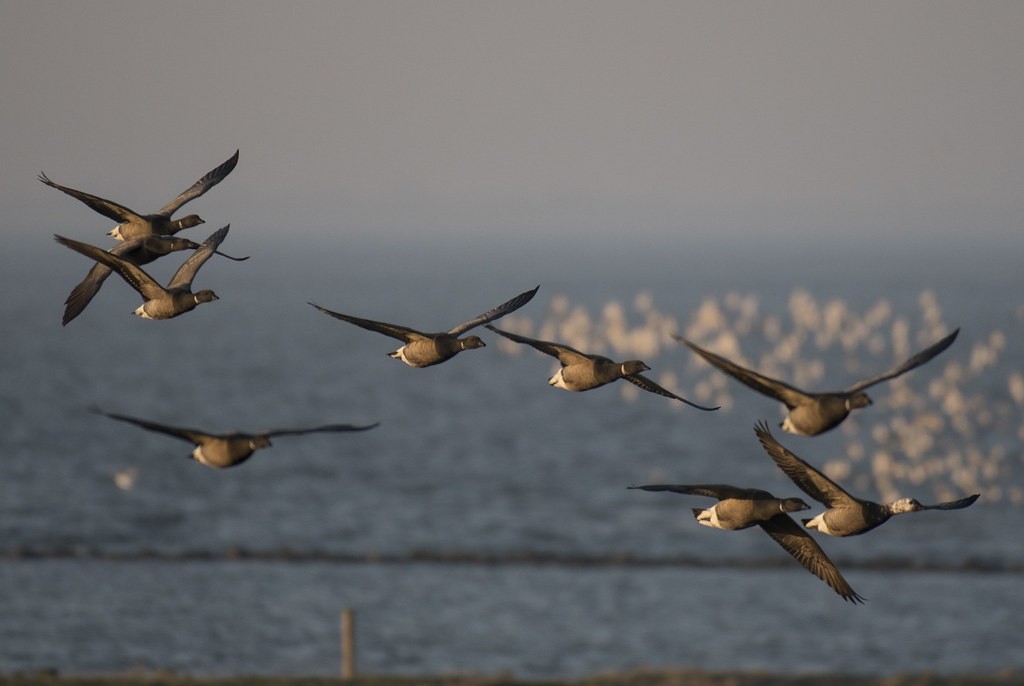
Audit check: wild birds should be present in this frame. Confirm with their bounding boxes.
[307,283,541,369]
[625,326,982,608]
[35,147,252,328]
[87,406,381,472]
[481,320,722,412]
